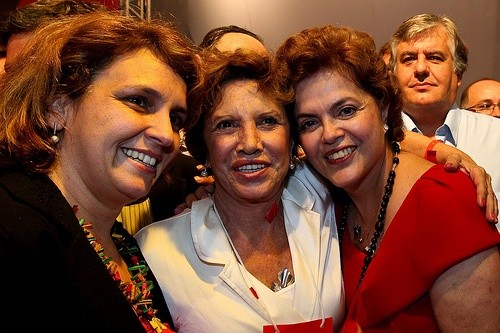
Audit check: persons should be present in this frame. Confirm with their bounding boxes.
[175,26,499,332]
[134,46,500,333]
[1,0,112,75]
[160,26,273,158]
[462,77,499,118]
[0,9,206,333]
[387,12,499,230]
[379,42,394,67]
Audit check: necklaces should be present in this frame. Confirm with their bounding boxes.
[69,206,167,332]
[336,138,401,294]
[352,207,383,253]
[212,196,325,331]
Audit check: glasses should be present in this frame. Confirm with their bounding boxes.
[465,101,500,111]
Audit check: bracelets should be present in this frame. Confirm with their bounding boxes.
[422,138,443,163]
